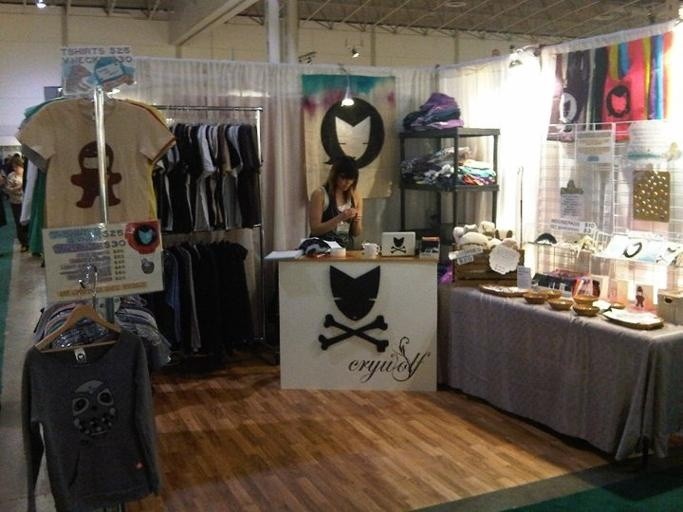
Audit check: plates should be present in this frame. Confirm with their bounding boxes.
[602,310,664,330]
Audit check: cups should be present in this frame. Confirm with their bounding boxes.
[365,243,380,258]
[331,247,346,258]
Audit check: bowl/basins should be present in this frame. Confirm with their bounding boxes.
[591,301,624,314]
[522,289,599,317]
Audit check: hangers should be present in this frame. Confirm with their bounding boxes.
[35,287,121,353]
[161,232,225,251]
[77,89,113,105]
[163,104,256,124]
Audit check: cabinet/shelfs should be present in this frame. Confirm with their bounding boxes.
[398,126,500,283]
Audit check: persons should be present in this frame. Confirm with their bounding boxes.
[307,157,363,248]
[4,156,29,253]
[0,168,8,257]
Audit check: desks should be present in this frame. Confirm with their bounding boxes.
[438,283,682,461]
[277,251,437,392]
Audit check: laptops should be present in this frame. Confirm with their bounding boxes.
[382,232,417,258]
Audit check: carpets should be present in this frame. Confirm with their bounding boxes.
[406,446,683,512]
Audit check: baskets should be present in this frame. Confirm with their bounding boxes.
[573,304,600,317]
[548,296,575,310]
[523,291,547,304]
[572,293,601,306]
[541,288,562,301]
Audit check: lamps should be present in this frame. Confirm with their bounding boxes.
[337,49,360,67]
[342,67,355,106]
